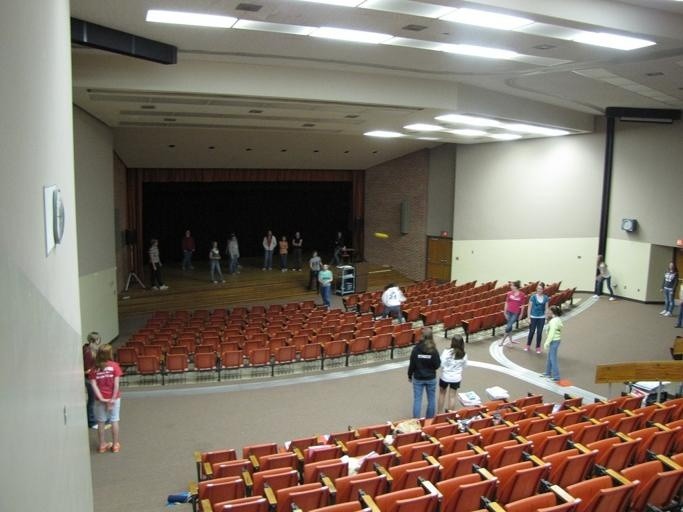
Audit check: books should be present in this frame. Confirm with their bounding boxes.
[486,386,509,401]
[457,390,481,406]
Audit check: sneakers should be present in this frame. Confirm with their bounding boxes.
[608,297,616,300]
[592,295,600,299]
[663,312,672,316]
[98,442,112,453]
[111,443,120,453]
[660,310,666,314]
[523,345,560,382]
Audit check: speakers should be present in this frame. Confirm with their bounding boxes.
[400,199,409,234]
[125,227,137,244]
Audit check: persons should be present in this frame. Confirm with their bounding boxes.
[539,306,563,383]
[659,261,678,317]
[307,230,346,310]
[83,332,122,453]
[499,282,524,347]
[438,334,466,414]
[181,230,195,270]
[148,239,168,290]
[523,280,550,354]
[378,281,407,324]
[595,255,617,302]
[260,229,304,272]
[208,233,240,284]
[407,327,441,419]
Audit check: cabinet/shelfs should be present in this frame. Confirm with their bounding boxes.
[334,265,356,296]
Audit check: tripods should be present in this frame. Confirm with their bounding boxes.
[125,247,146,293]
